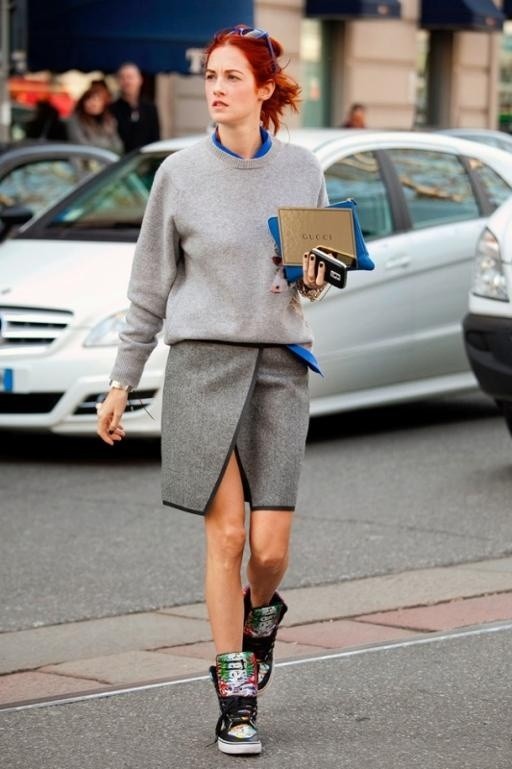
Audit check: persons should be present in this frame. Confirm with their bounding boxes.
[499,101,511,134]
[26,63,162,191]
[96,25,331,759]
[339,104,367,127]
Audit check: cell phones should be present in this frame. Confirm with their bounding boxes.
[316,245,356,271]
[309,248,347,289]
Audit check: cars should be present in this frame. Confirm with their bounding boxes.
[461,195,511,430]
[0,134,149,238]
[0,124,509,450]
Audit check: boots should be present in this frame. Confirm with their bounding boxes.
[241,585,288,696]
[204,649,263,754]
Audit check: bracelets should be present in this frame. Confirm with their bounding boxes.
[108,380,132,393]
[297,279,325,303]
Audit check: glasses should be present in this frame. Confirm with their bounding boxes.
[232,24,276,67]
